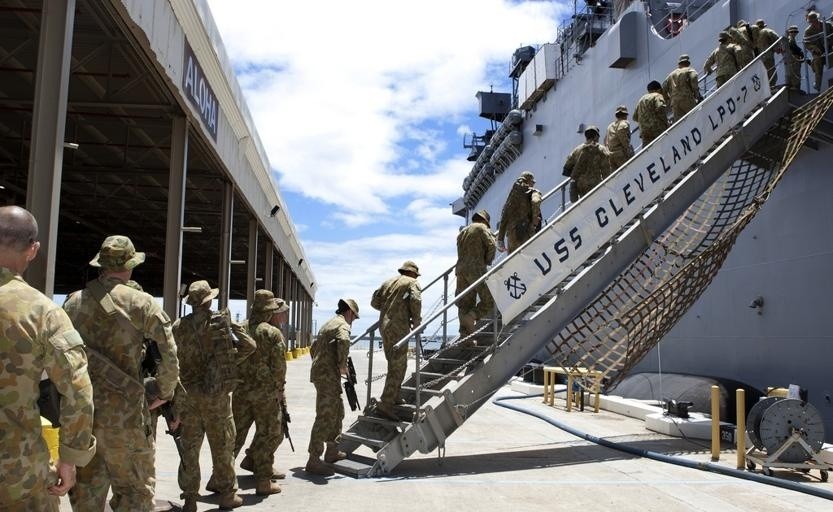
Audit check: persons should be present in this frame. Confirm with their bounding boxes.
[563,124,611,205]
[454,208,503,348]
[662,53,704,124]
[239,298,287,480]
[632,79,672,149]
[60,233,181,512]
[495,170,544,256]
[602,104,635,170]
[371,261,423,423]
[303,298,361,477]
[0,202,99,512]
[160,279,258,512]
[205,289,287,495]
[702,10,833,95]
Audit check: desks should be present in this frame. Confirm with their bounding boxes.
[542,364,601,413]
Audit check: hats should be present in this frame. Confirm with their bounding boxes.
[718,19,767,42]
[398,260,420,276]
[614,105,628,115]
[339,298,359,318]
[584,126,600,137]
[787,26,798,32]
[475,210,490,228]
[87,235,290,316]
[677,55,690,65]
[806,12,819,22]
[518,172,536,183]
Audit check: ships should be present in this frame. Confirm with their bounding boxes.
[450,0,832,448]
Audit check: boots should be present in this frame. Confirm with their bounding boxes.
[396,396,406,404]
[460,310,476,331]
[182,453,285,511]
[376,398,398,421]
[305,455,334,475]
[324,446,347,463]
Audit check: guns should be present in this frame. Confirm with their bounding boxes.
[344,357,361,411]
[142,343,190,470]
[280,402,295,454]
